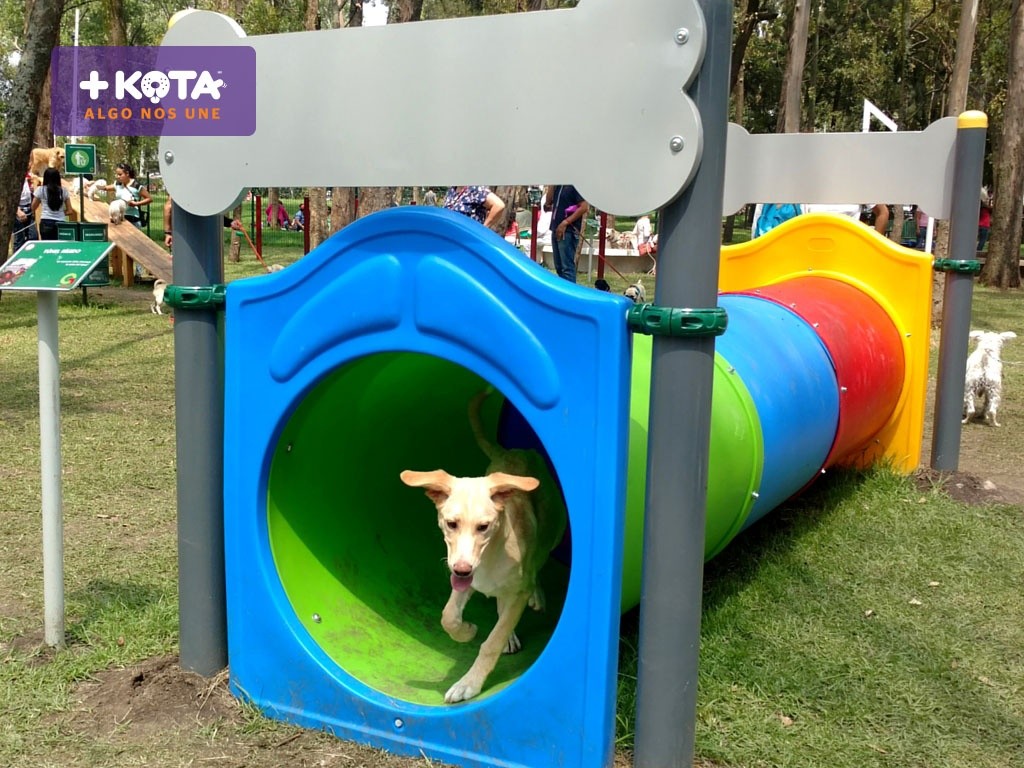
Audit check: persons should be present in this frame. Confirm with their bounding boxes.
[423,187,437,207]
[524,185,552,266]
[633,214,655,274]
[504,212,520,249]
[750,204,801,240]
[976,197,993,251]
[10,160,305,256]
[544,185,589,285]
[442,185,506,229]
[915,208,938,248]
[801,204,890,236]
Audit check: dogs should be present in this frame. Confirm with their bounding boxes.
[109,199,128,225]
[961,328,1018,427]
[30,147,65,179]
[150,279,172,316]
[399,393,568,703]
[266,264,284,274]
[624,279,647,303]
[606,228,633,249]
[73,176,107,202]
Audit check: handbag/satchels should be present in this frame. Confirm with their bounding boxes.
[121,181,147,227]
